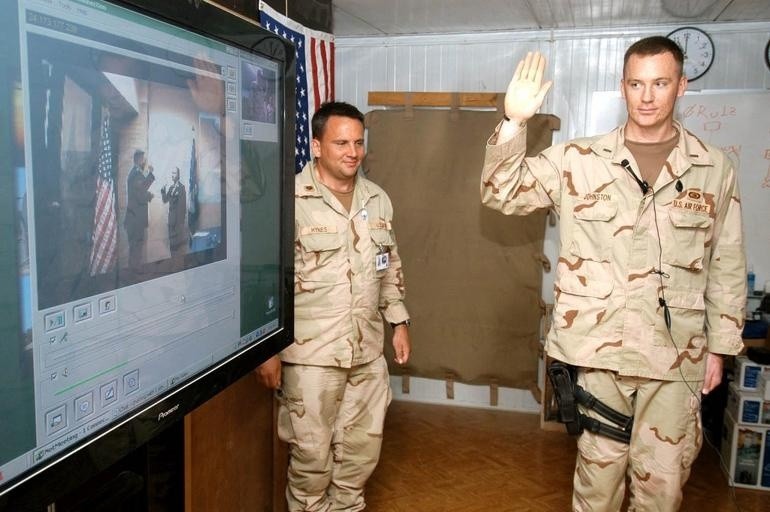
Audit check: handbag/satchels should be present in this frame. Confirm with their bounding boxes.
[547,360,584,434]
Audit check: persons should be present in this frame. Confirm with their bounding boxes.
[480,37,748,512]
[254,102,412,512]
[123,150,156,266]
[160,167,186,257]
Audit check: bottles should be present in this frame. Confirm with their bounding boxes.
[747,264,755,296]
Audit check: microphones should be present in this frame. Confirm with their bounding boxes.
[621,159,647,193]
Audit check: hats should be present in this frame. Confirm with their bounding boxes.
[756,293,770,314]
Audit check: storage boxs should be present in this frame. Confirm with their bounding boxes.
[715,355,769,492]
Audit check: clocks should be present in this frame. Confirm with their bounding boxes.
[664,26,715,83]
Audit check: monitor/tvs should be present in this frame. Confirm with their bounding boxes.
[0,2,295,512]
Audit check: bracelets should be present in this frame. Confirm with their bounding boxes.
[504,115,527,127]
[391,319,411,329]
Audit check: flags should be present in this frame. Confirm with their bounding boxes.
[258,1,334,175]
[188,140,199,226]
[87,105,118,275]
